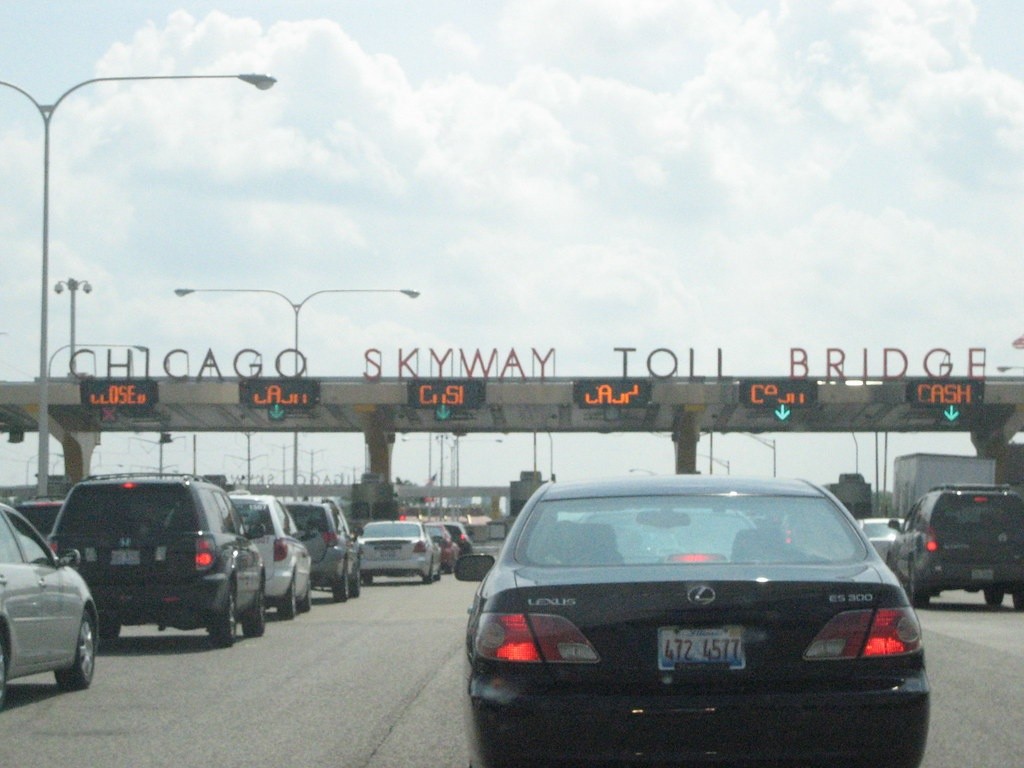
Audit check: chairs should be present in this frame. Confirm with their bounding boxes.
[539,519,616,570]
[730,525,791,566]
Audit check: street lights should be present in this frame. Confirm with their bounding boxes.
[2,72,278,498]
[54,278,94,377]
[172,288,422,502]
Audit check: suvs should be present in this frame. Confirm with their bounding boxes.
[47,470,271,650]
[281,497,363,602]
[888,484,1023,615]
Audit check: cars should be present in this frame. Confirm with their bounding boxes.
[19,501,62,543]
[1,499,101,701]
[354,519,446,586]
[229,493,313,621]
[459,471,933,767]
[445,523,475,559]
[424,522,458,573]
[855,518,907,570]
